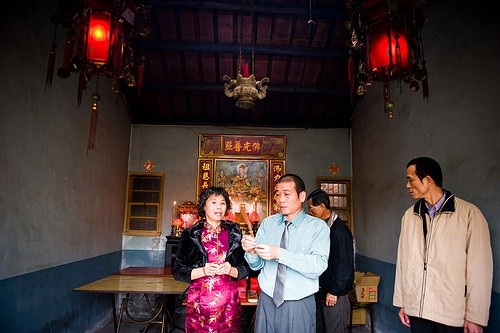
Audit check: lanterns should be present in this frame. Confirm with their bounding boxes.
[346,0,430,118]
[46,0,123,150]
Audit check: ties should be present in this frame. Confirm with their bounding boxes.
[272,220,292,308]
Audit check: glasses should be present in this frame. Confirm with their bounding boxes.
[308,205,321,212]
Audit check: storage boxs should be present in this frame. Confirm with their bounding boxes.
[352,305,367,325]
[352,272,381,303]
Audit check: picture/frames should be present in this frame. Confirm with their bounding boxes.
[214,158,271,225]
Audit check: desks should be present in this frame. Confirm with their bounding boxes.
[182,297,258,333]
[165,235,181,267]
[73,267,190,333]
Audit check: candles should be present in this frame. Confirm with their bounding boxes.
[172,201,177,222]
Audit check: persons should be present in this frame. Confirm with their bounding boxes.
[393,156,493,333]
[170,186,249,333]
[241,175,330,333]
[306,190,353,333]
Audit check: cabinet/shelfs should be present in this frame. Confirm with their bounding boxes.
[348,296,381,333]
